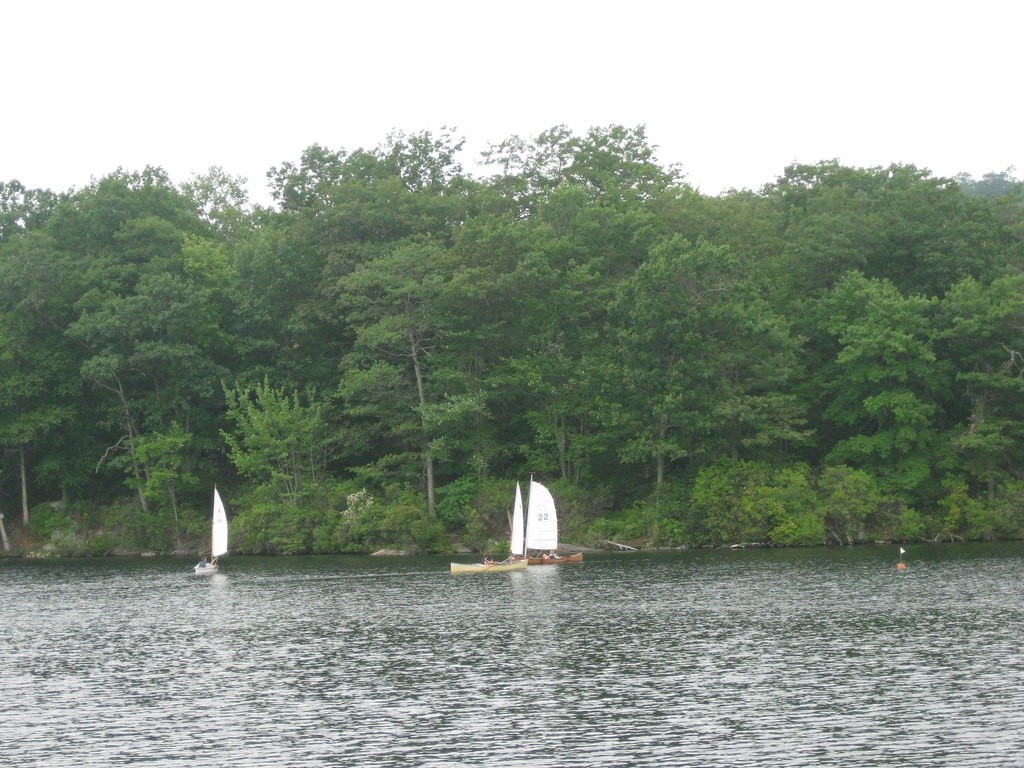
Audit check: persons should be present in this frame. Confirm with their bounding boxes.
[483,555,497,564]
[199,556,206,567]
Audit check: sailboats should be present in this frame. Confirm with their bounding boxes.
[450,480,528,577]
[481,478,584,566]
[195,484,228,575]
